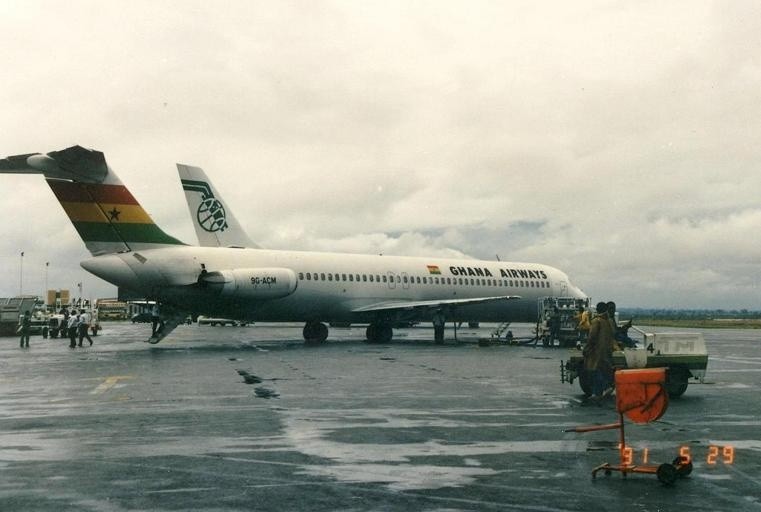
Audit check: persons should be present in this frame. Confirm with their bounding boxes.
[550,307,562,347]
[572,307,592,340]
[20,310,31,348]
[68,310,78,348]
[584,301,616,404]
[77,309,94,346]
[433,310,447,344]
[606,301,638,348]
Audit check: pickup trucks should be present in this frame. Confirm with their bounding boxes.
[132,312,155,322]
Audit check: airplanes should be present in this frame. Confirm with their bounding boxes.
[0,146,598,347]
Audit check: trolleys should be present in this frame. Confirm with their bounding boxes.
[562,364,701,484]
